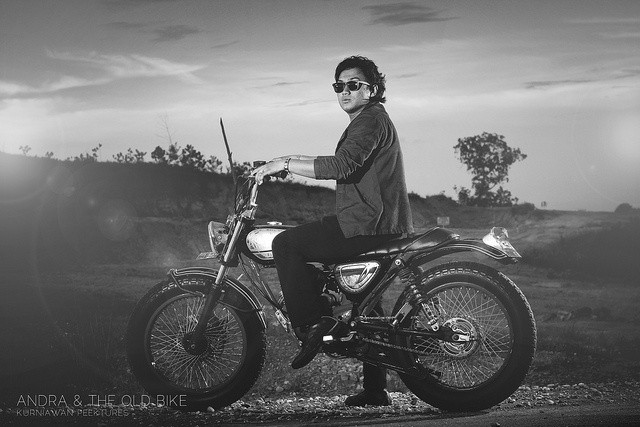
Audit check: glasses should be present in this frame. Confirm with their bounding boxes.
[332,80,371,93]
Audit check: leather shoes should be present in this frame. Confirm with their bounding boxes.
[292,316,339,370]
[345,390,391,406]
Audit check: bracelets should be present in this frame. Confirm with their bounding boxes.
[296,154,301,160]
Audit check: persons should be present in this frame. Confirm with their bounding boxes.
[251,56,415,406]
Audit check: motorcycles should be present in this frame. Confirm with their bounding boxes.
[126,161,537,412]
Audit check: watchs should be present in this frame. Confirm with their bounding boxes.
[284,157,291,175]
